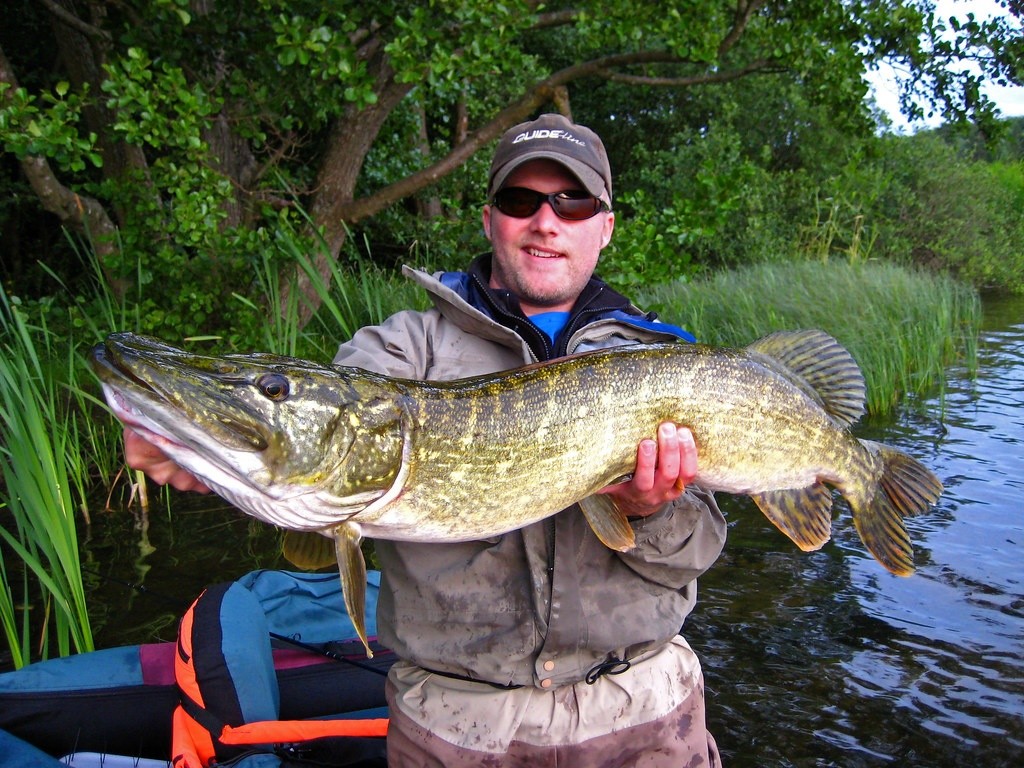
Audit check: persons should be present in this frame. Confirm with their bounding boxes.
[123,113,728,768]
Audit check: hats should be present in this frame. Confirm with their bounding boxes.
[488,114,613,209]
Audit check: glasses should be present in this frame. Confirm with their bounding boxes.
[491,185,602,220]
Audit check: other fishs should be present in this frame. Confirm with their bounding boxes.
[91,326,946,661]
